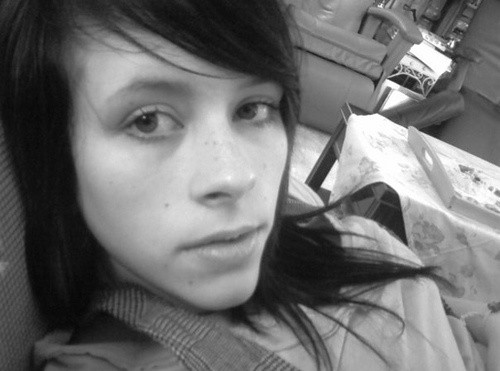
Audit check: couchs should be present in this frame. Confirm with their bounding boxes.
[279,0,422,135]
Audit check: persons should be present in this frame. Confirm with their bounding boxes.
[1,0,500,371]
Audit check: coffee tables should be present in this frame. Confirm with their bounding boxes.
[305,102,500,247]
